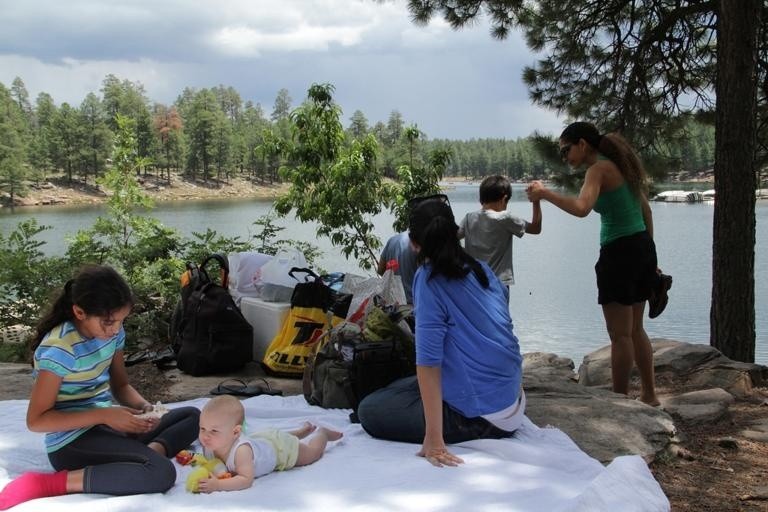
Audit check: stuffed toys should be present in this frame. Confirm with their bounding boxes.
[187,453,234,495]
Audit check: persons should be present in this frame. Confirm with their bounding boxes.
[0,263,202,511]
[194,391,346,494]
[523,121,663,410]
[456,175,544,291]
[354,193,529,471]
[375,226,423,317]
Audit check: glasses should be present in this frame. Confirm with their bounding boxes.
[560,144,572,157]
[408,194,450,221]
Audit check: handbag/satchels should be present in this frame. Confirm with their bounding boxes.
[303,328,410,423]
[262,267,353,377]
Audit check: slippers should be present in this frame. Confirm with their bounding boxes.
[247,378,282,395]
[211,379,262,396]
[123,341,174,366]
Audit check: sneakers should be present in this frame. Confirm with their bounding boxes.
[649,274,672,318]
